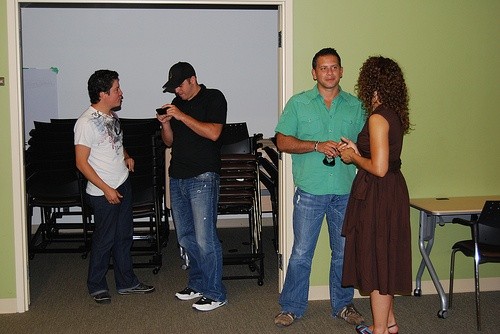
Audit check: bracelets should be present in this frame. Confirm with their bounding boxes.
[314,141,320,152]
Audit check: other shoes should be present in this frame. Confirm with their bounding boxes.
[356,323,399,334]
[118,283,154,294]
[94,292,111,301]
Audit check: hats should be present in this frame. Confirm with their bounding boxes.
[162,62,196,88]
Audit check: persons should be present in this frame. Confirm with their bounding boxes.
[335,56,412,334]
[272,48,368,329]
[74,69,156,304]
[156,62,229,311]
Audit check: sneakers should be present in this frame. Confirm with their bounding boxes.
[274,313,294,326]
[192,297,227,311]
[337,304,365,324]
[175,287,202,300]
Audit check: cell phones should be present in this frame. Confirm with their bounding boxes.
[156,108,166,115]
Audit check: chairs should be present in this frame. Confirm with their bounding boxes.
[448,200,500,331]
[23,119,279,286]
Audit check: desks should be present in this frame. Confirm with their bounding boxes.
[409,196,500,318]
